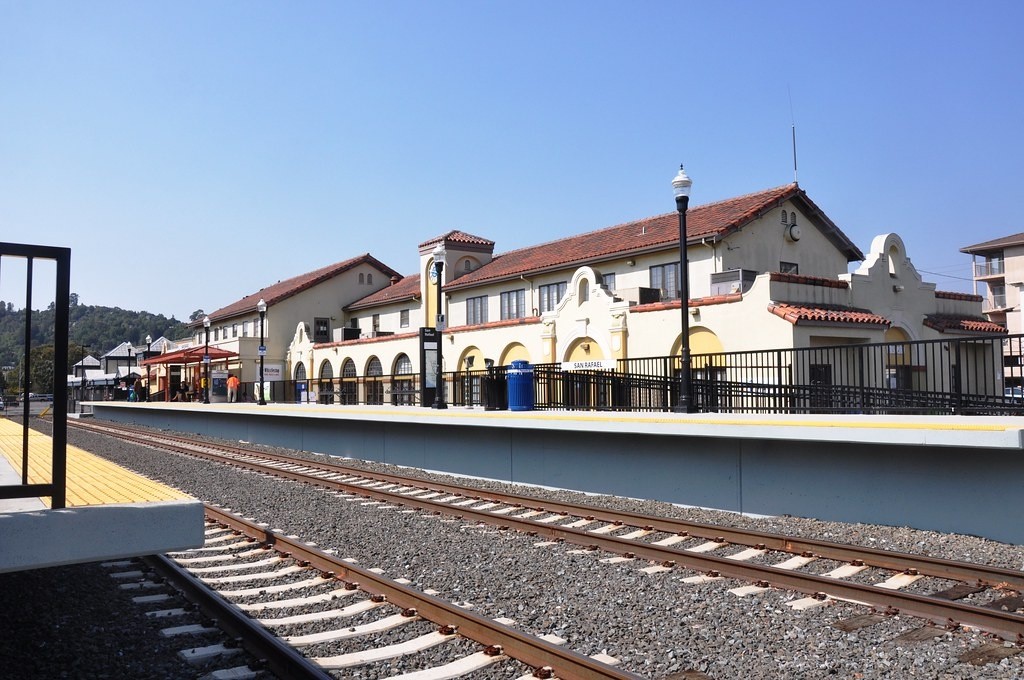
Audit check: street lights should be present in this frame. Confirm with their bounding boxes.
[433,243,446,409]
[19,355,24,398]
[126,341,132,400]
[257,298,267,404]
[146,335,152,400]
[203,315,211,404]
[81,344,91,402]
[671,162,696,413]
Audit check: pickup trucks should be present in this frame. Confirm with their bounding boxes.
[20,393,48,402]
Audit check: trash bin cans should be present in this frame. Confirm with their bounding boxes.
[485,366,508,410]
[506,361,535,411]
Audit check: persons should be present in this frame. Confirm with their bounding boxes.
[133,378,146,402]
[201,371,210,403]
[225,376,240,402]
[171,382,194,402]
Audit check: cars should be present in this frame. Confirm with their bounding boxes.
[47,394,53,401]
[1005,387,1024,403]
[0,397,4,410]
